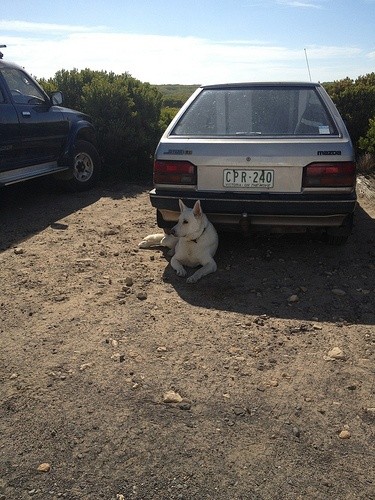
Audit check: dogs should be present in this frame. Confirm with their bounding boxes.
[138,199,219,284]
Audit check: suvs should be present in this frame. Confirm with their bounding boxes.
[0,51,102,199]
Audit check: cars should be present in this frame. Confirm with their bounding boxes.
[148,79,358,245]
